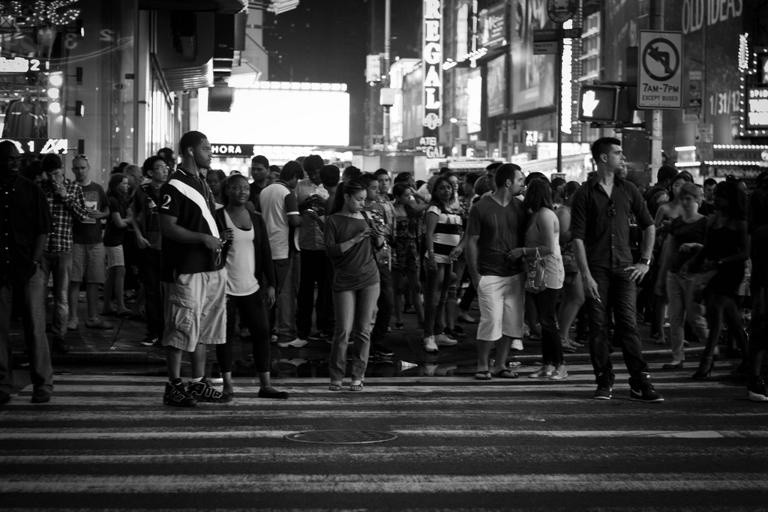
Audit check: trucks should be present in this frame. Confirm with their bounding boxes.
[432,155,503,199]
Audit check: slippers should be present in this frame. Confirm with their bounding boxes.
[349,381,363,391]
[329,384,341,391]
[491,368,519,379]
[474,367,492,380]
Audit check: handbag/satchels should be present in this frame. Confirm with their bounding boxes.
[523,247,546,293]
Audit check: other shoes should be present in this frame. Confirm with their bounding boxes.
[58,307,162,347]
[270,308,460,369]
[239,328,252,337]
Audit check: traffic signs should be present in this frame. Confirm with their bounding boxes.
[534,28,582,42]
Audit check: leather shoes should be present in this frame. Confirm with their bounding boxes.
[455,313,478,324]
[662,360,683,370]
[257,387,289,399]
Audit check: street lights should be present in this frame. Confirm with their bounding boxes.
[379,86,395,147]
[448,117,490,157]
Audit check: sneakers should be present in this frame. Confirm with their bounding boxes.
[186,375,234,403]
[32,384,53,403]
[527,318,690,381]
[630,383,665,403]
[592,383,613,400]
[746,376,768,402]
[163,378,198,408]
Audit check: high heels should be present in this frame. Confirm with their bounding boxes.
[692,358,715,380]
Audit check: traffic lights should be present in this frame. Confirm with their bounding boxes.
[580,85,618,122]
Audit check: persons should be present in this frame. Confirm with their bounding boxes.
[0,132,768,407]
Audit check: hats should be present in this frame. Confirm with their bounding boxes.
[427,174,446,194]
[681,182,698,196]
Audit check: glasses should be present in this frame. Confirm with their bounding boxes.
[154,166,170,169]
